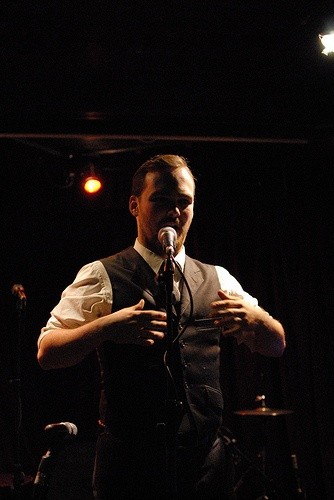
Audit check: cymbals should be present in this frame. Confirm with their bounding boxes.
[234,407,295,416]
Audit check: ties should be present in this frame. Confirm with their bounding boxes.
[163,260,180,305]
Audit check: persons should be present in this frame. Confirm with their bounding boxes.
[35,153,287,500]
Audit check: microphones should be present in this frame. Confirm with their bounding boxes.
[158,227,177,254]
[12,284,26,301]
[44,422,78,440]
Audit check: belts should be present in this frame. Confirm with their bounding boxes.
[159,431,218,448]
